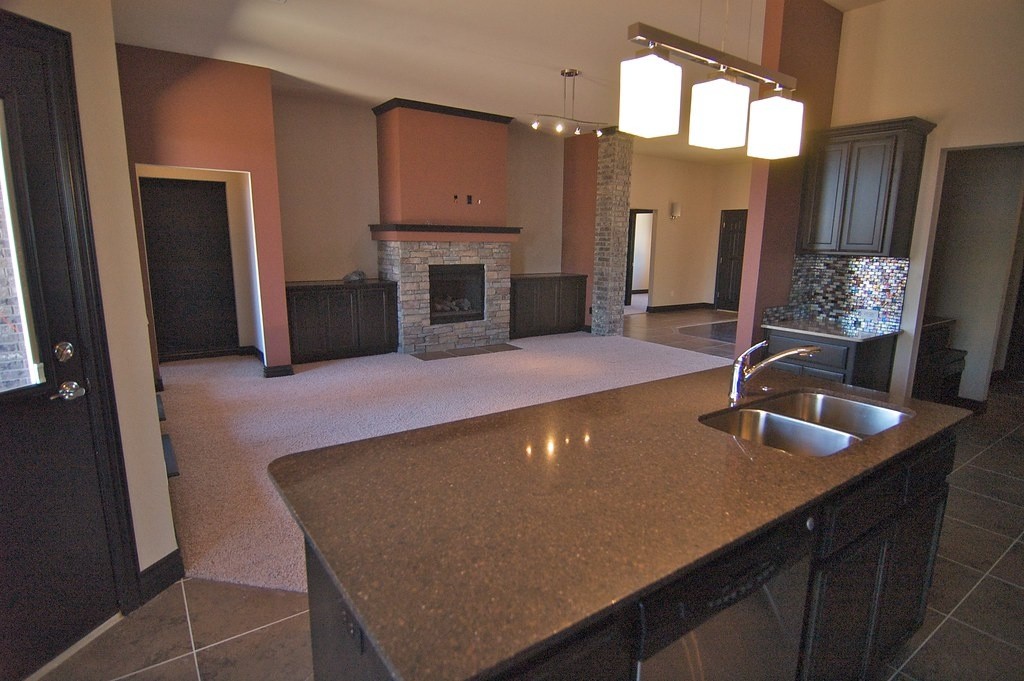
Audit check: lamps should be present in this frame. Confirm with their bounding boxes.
[618,0,804,160]
[529,69,609,138]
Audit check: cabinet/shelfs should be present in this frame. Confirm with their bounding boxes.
[285,278,398,365]
[762,319,904,393]
[912,315,956,404]
[509,272,589,339]
[794,116,937,257]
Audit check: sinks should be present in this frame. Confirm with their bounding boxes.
[697,407,863,459]
[758,389,913,440]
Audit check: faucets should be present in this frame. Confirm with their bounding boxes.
[728,338,825,403]
